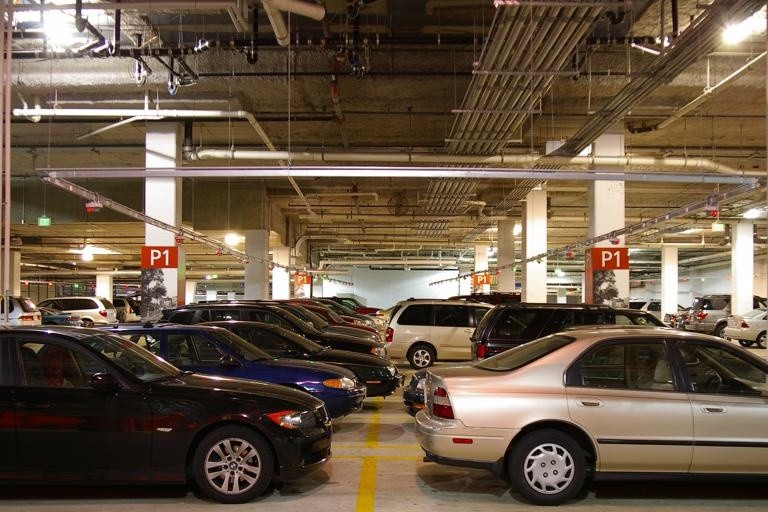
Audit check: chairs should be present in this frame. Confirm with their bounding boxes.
[650,359,677,391]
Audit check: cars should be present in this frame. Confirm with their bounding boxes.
[0,325,333,504]
[402,361,486,418]
[414,324,767,506]
[93,321,366,419]
